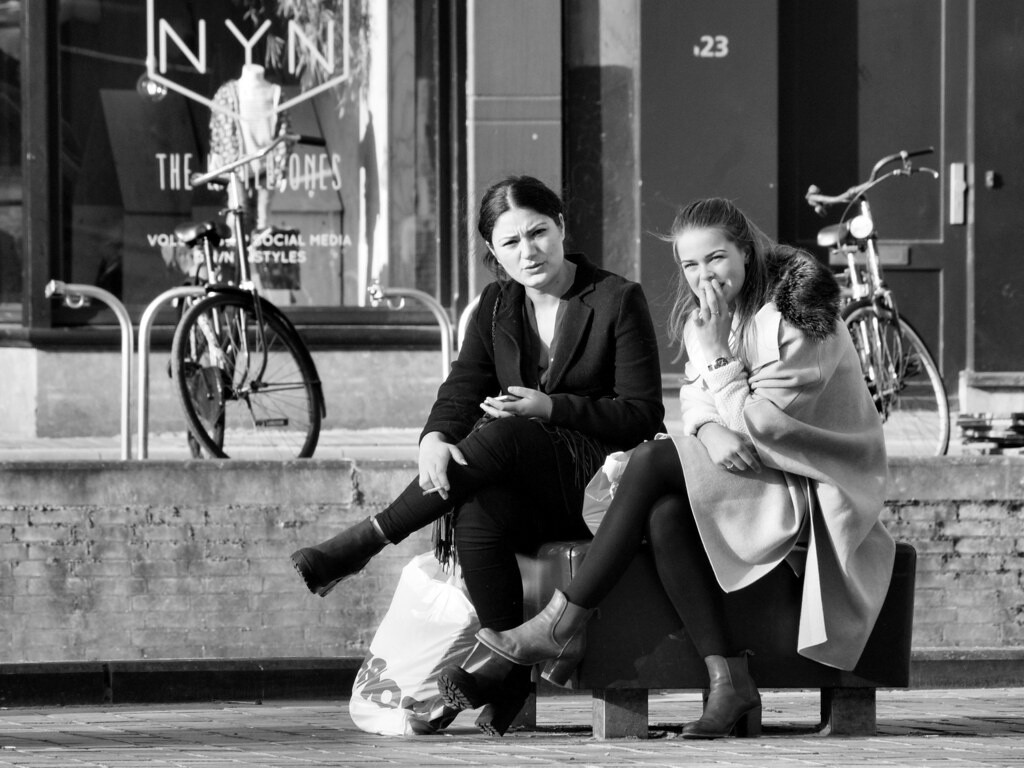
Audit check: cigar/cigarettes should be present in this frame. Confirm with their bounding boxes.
[422,485,442,497]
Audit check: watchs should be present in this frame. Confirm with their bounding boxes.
[704,356,738,373]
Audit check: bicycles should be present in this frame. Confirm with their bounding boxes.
[803,147,951,457]
[166,132,330,458]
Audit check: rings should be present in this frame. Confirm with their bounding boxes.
[726,463,734,469]
[710,310,720,317]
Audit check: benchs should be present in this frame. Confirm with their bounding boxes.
[515,541,915,739]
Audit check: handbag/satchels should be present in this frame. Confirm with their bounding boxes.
[348,547,480,738]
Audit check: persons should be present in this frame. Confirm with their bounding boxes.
[290,174,666,738]
[207,64,295,292]
[471,198,898,737]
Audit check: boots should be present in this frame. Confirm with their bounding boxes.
[478,587,602,688]
[684,652,763,741]
[438,652,531,738]
[289,514,388,598]
